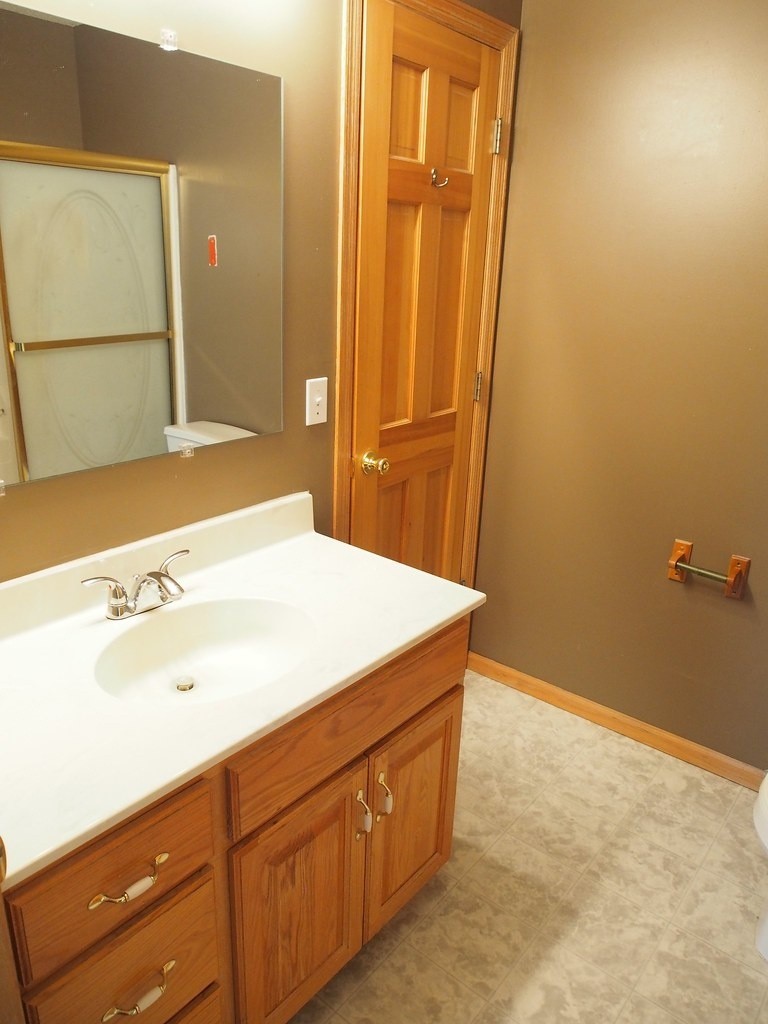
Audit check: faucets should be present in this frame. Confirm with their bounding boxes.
[129,570,186,618]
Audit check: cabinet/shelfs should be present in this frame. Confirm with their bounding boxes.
[0,615,473,1024]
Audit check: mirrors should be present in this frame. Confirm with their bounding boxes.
[0,1,284,490]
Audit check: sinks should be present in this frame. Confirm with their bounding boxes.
[93,566,317,721]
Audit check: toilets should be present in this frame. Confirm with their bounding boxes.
[163,419,257,452]
